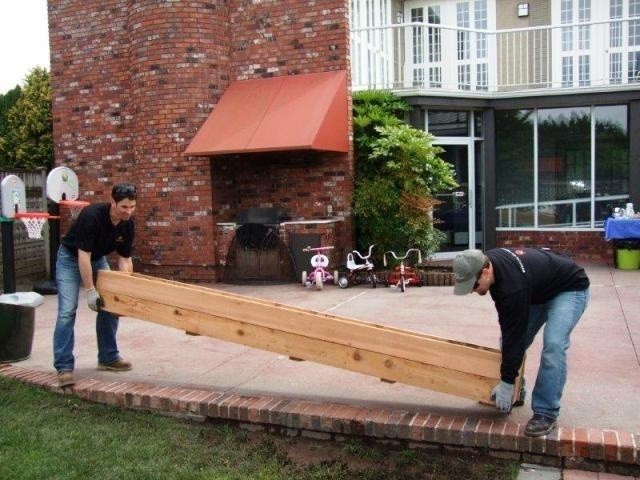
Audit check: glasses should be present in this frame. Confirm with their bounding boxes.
[116,185,138,195]
[474,271,483,289]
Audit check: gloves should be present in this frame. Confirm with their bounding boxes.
[85,287,103,310]
[491,380,515,411]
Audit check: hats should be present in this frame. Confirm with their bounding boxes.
[451,248,485,295]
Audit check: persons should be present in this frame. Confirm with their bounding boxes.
[452,246,590,437]
[51,181,138,386]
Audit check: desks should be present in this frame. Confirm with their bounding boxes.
[605,217,640,269]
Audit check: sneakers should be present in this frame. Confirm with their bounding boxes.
[56,370,75,388]
[512,386,525,407]
[524,413,558,436]
[97,358,132,372]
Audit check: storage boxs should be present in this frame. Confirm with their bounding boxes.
[617,249,640,269]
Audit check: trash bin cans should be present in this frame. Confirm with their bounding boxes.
[0,302,35,363]
[615,238,640,270]
